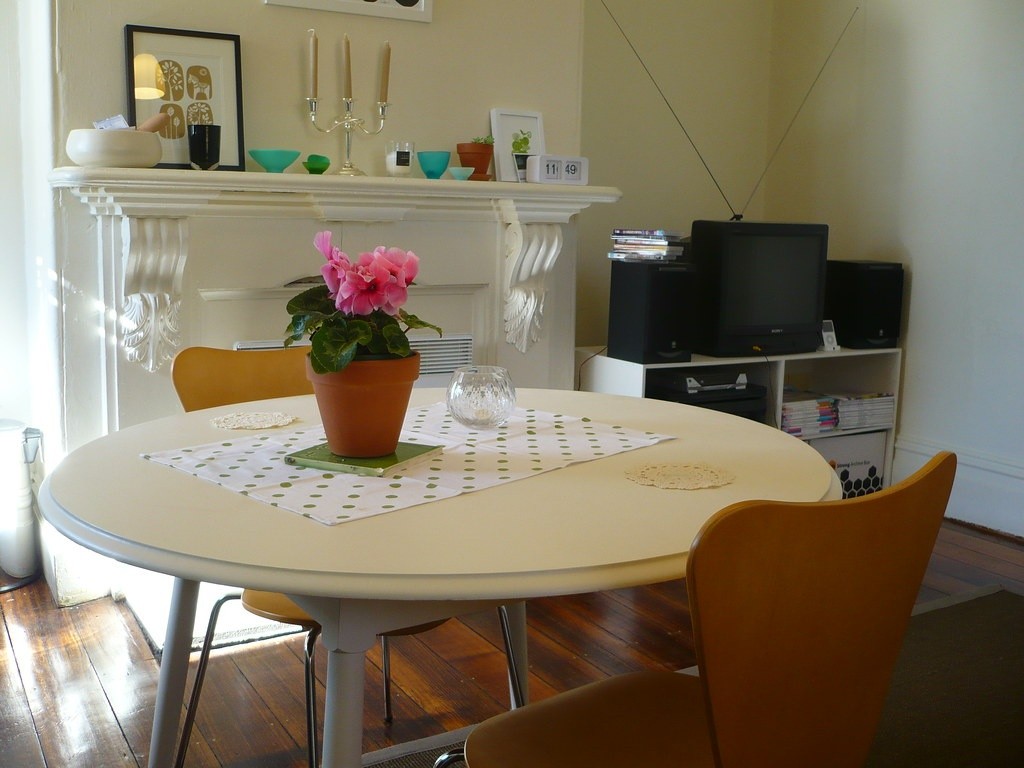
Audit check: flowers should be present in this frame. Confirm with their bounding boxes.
[280,227,442,374]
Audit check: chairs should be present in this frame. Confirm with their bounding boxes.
[171,346,525,768]
[462,450,957,768]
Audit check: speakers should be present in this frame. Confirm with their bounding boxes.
[607,261,692,364]
[827,260,904,349]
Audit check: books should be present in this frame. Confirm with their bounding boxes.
[782,389,894,439]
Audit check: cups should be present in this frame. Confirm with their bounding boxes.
[384,139,415,178]
[446,365,517,430]
[188,124,221,171]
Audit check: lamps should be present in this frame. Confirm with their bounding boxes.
[133,53,166,100]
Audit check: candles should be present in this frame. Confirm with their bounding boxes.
[380,41,392,103]
[307,29,318,99]
[341,33,352,98]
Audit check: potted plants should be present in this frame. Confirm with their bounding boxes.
[457,134,495,181]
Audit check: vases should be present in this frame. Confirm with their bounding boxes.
[306,352,421,457]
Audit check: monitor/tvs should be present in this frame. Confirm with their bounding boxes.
[691,220,828,357]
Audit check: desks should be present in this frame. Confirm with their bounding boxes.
[36,383,844,768]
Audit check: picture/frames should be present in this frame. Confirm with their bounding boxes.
[124,24,246,171]
[491,107,545,183]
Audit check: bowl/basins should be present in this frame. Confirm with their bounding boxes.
[248,148,302,173]
[303,161,330,174]
[65,128,162,169]
[448,166,475,180]
[417,150,451,179]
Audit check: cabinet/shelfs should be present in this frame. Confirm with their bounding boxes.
[576,342,903,489]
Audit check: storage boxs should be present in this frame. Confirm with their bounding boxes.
[807,430,888,500]
[656,391,766,426]
